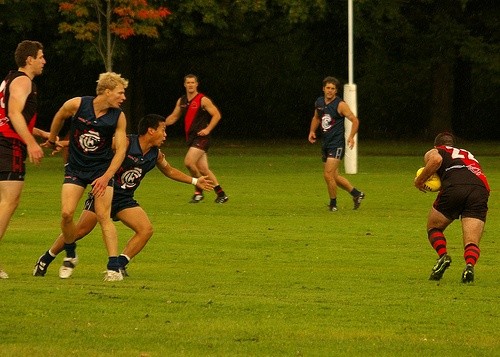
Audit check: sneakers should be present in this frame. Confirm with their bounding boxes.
[0,270,8,278]
[121,268,129,278]
[352,192,365,210]
[462,267,474,283]
[190,194,204,204]
[59,256,78,279]
[105,268,123,283]
[329,206,339,212]
[33,255,48,276]
[429,255,452,280]
[214,195,228,203]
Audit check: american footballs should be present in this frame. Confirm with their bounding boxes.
[416,167,442,192]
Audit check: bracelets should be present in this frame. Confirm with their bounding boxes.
[48,139,55,145]
[192,177,198,185]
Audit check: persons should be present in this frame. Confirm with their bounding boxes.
[0,40,47,278]
[308,76,364,212]
[33,114,215,277]
[40,72,129,279]
[414,132,491,284]
[164,73,229,204]
